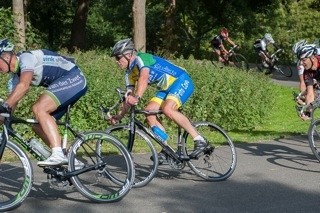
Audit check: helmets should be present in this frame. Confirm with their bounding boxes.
[221,30,228,39]
[292,40,308,55]
[265,33,272,42]
[0,38,14,55]
[110,38,134,57]
[297,44,316,60]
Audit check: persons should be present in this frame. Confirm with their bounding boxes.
[292,38,320,119]
[253,33,281,67]
[0,40,87,169]
[210,28,240,64]
[107,38,208,163]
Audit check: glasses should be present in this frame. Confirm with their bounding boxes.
[116,52,130,61]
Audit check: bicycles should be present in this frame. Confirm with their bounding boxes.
[255,49,293,77]
[0,105,135,211]
[295,99,320,163]
[211,45,250,70]
[95,88,238,188]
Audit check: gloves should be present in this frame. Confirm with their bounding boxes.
[0,102,10,117]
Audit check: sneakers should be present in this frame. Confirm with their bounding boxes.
[150,151,178,163]
[63,159,84,186]
[36,151,68,166]
[189,138,210,160]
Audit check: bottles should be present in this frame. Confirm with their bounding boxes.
[27,137,53,158]
[151,124,168,141]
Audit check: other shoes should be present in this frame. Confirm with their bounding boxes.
[262,62,269,67]
